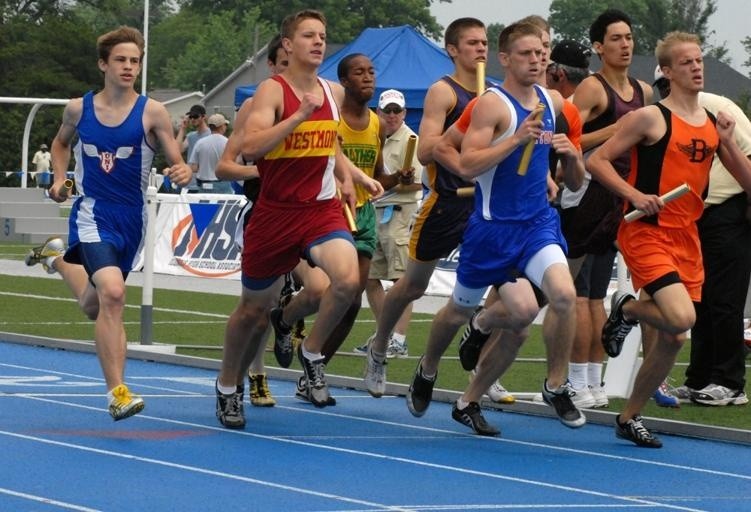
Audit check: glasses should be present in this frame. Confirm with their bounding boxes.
[189,114,201,118]
[657,79,668,91]
[381,108,403,114]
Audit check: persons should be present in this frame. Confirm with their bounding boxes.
[405,21,588,429]
[188,113,241,193]
[352,87,425,358]
[212,9,362,429]
[651,62,749,407]
[31,143,51,189]
[583,30,750,446]
[215,8,657,413]
[175,103,211,192]
[293,52,415,406]
[24,24,193,421]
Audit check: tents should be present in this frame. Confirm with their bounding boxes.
[233,23,503,135]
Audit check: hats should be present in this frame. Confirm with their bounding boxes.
[208,114,230,128]
[378,89,406,110]
[186,105,206,115]
[548,39,592,69]
[652,63,664,87]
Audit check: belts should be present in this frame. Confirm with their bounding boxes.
[376,205,402,211]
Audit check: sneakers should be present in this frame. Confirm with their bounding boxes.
[216,377,247,429]
[364,341,388,397]
[533,385,598,408]
[615,414,662,448]
[601,290,640,357]
[291,329,306,356]
[468,367,515,404]
[354,343,368,353]
[108,384,145,421]
[294,376,337,406]
[665,386,695,403]
[542,378,587,428]
[688,383,749,406]
[588,382,610,408]
[238,384,246,423]
[268,307,299,368]
[297,336,329,408]
[459,306,494,371]
[281,271,301,296]
[452,399,501,436]
[26,236,64,274]
[386,336,409,359]
[407,354,438,417]
[280,293,305,339]
[248,369,277,406]
